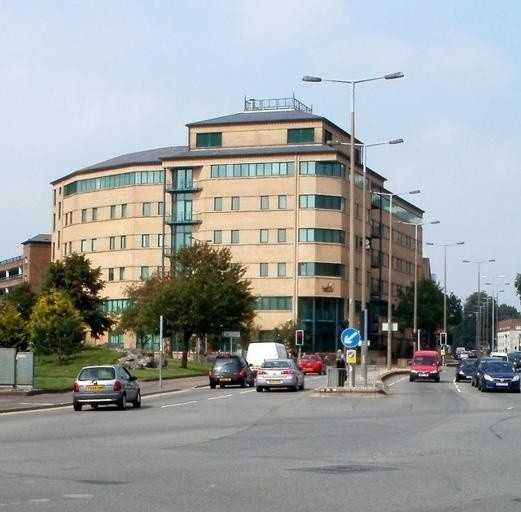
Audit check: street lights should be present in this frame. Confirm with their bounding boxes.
[326,138,403,375]
[393,220,440,354]
[462,257,510,357]
[367,189,420,369]
[302,72,404,385]
[425,241,466,332]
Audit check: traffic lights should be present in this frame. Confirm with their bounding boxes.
[440,333,447,345]
[296,330,303,345]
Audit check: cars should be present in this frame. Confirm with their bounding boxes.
[209,352,329,392]
[455,347,521,392]
[72,365,141,410]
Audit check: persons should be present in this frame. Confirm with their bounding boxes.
[336,347,347,386]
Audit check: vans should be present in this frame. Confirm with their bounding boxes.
[246,342,288,371]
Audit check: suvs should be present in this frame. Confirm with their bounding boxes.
[407,350,441,382]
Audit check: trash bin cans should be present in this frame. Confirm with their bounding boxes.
[398,359,407,369]
